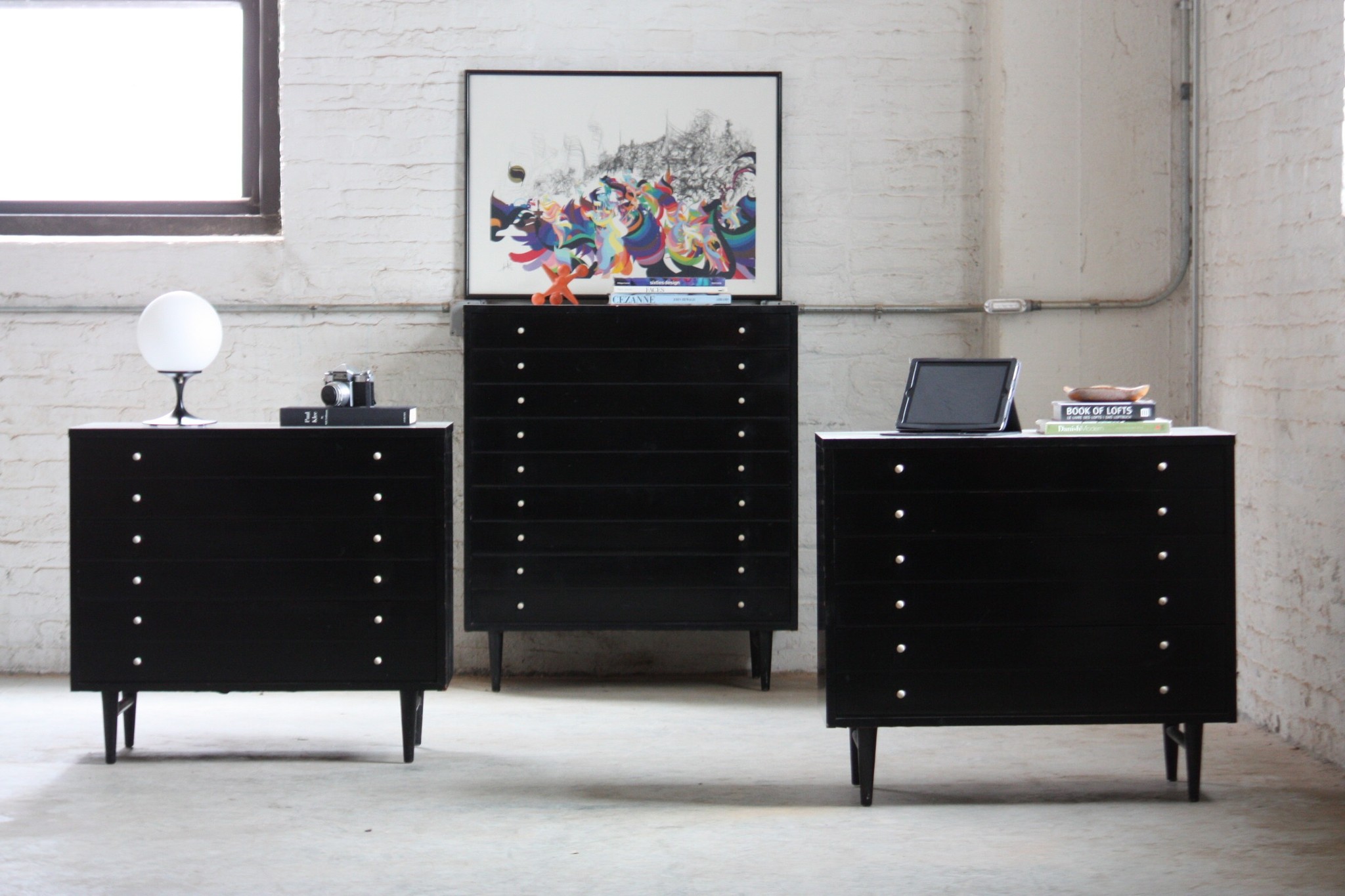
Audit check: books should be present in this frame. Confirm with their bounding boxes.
[279,404,418,426]
[609,276,732,306]
[1036,401,1171,435]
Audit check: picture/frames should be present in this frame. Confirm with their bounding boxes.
[465,68,780,302]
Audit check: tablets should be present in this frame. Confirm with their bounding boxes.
[895,357,1020,432]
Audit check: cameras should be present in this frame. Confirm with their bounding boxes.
[320,370,376,408]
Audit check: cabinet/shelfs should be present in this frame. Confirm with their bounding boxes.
[816,426,1239,810]
[465,299,800,696]
[68,421,454,763]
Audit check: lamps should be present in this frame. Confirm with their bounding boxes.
[138,291,223,426]
